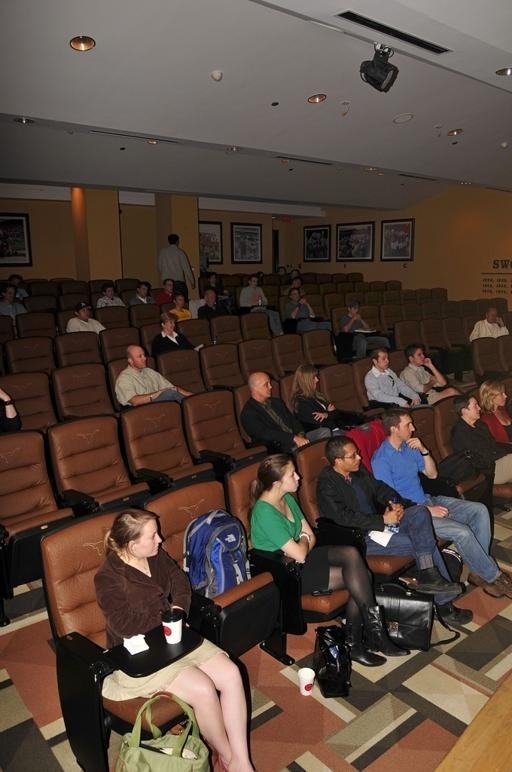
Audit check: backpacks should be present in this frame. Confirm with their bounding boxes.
[182,509,252,598]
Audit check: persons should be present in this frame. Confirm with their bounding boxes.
[158,234,196,304]
[388,228,409,254]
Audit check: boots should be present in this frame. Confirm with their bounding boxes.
[342,618,386,667]
[363,604,409,656]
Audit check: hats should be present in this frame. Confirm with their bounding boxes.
[74,302,90,312]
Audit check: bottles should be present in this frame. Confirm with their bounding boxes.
[388,493,400,533]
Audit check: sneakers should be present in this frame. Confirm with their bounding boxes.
[417,565,462,594]
[433,605,473,625]
[468,572,512,599]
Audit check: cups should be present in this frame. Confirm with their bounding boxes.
[297,667,316,697]
[160,608,184,646]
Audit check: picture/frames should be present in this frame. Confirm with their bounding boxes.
[380,217,415,262]
[1,212,33,268]
[301,223,332,265]
[230,222,262,264]
[335,222,374,262]
[198,220,224,265]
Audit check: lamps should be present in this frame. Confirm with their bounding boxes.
[357,42,400,94]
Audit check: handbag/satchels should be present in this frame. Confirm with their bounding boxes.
[440,546,463,581]
[115,734,211,772]
[436,450,486,484]
[314,625,353,698]
[372,586,435,652]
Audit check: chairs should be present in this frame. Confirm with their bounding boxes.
[0,273,510,772]
